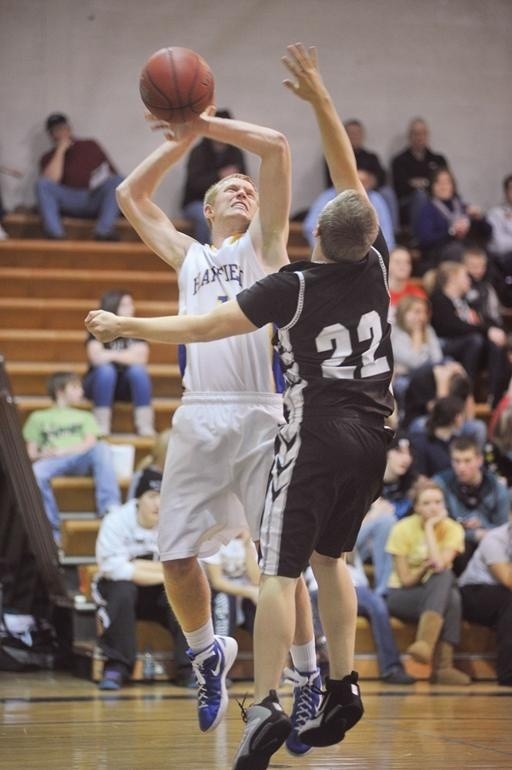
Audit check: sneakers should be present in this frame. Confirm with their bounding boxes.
[285,668,362,757]
[99,670,122,691]
[381,670,415,684]
[187,634,239,733]
[231,689,291,769]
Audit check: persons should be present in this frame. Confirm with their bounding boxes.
[34,115,126,242]
[182,111,246,243]
[0,292,293,691]
[84,43,393,768]
[116,103,327,753]
[307,118,511,690]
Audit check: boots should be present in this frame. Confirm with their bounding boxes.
[406,611,445,665]
[433,640,472,684]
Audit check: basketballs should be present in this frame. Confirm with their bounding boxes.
[139,46,216,124]
